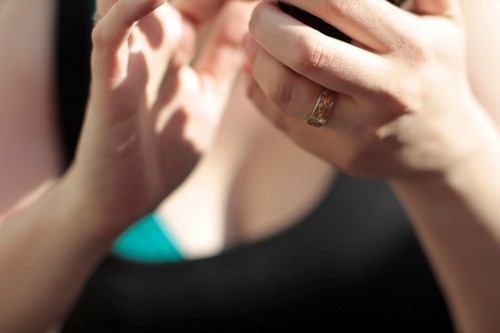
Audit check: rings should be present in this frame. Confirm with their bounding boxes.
[306,87,338,127]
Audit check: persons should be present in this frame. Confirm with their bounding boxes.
[0,0,500,333]
